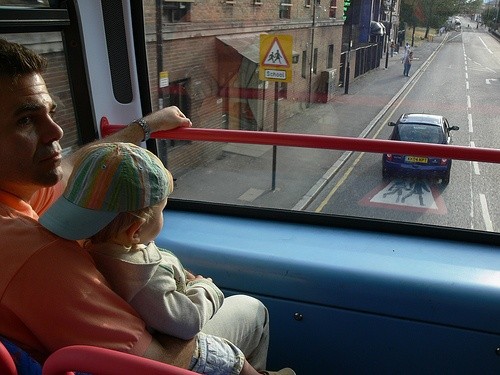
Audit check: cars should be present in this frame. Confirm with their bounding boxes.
[382,113,460,184]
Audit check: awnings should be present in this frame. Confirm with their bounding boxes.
[370,21,389,36]
[214,30,301,97]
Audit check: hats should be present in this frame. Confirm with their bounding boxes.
[38,141,174,239]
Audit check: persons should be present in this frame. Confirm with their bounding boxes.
[0,35,269,375]
[40,142,297,375]
[402,42,413,77]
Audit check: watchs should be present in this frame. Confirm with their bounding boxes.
[128,119,150,142]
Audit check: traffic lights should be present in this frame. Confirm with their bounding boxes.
[342,0,351,27]
